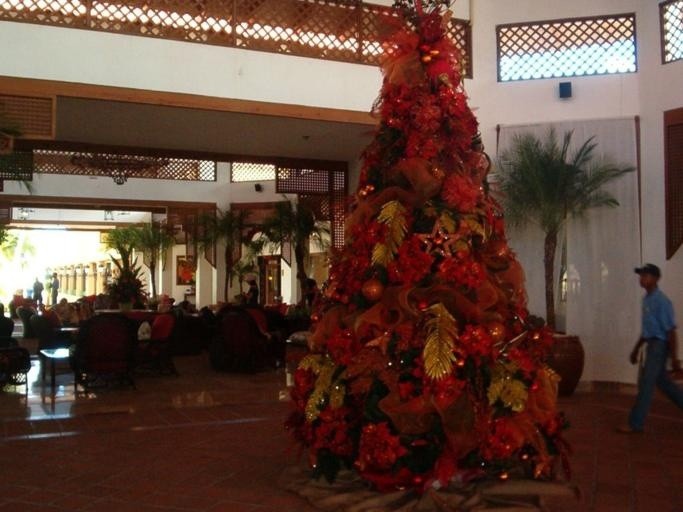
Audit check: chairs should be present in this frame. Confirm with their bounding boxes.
[0,296,289,389]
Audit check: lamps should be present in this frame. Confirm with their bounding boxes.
[71,151,170,185]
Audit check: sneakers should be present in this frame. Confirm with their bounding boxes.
[616,422,636,432]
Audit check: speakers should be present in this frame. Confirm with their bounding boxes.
[254,183,261,192]
[559,82,573,98]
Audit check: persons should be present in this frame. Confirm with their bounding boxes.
[614,262,683,433]
[1,273,259,386]
[297,271,318,308]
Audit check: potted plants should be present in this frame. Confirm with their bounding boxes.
[491,126,636,394]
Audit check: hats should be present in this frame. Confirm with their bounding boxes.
[633,263,660,277]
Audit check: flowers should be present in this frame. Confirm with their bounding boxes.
[106,271,145,302]
